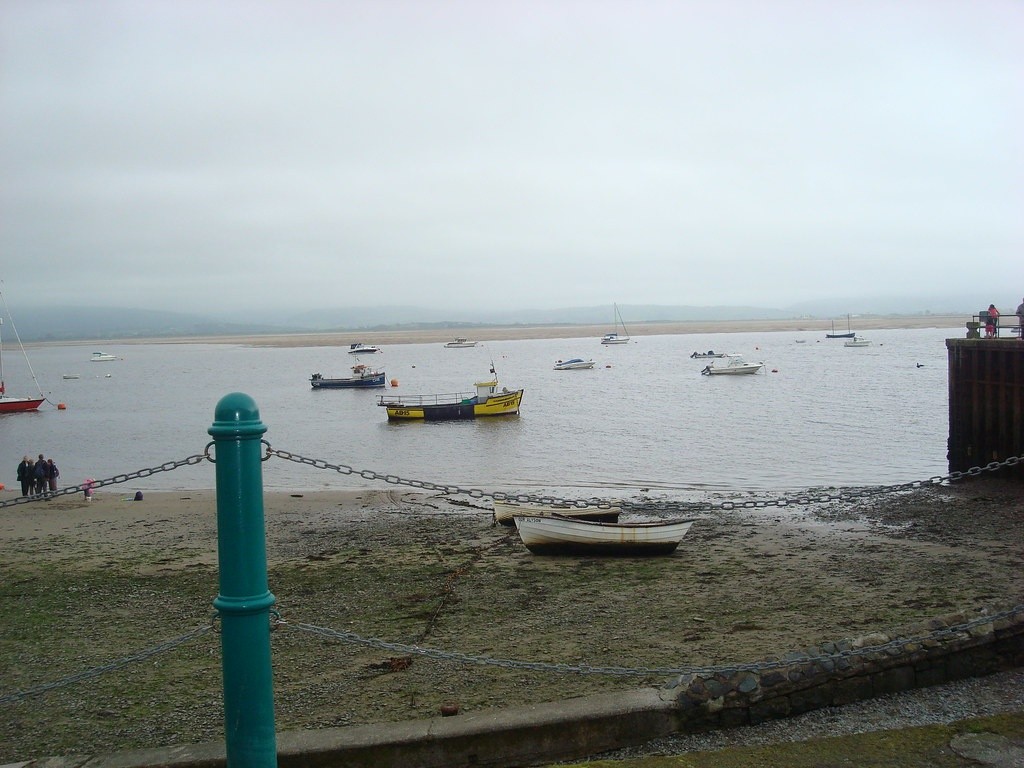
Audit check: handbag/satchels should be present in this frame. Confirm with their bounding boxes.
[54,467,59,477]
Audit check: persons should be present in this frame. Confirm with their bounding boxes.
[17,454,59,496]
[985,304,999,337]
[1016,298,1024,340]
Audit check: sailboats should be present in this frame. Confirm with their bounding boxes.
[0,289,48,413]
[602,302,630,345]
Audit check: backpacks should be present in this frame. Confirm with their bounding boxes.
[34,461,46,478]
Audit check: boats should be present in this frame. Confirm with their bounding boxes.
[691,349,765,375]
[825,313,856,338]
[376,346,526,420]
[443,337,479,348]
[308,354,385,388]
[843,335,872,347]
[347,342,381,354]
[89,351,118,361]
[552,357,595,370]
[492,499,698,556]
